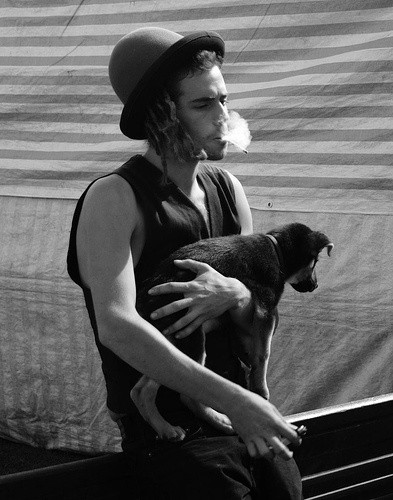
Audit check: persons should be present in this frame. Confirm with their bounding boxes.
[67,27,302,496]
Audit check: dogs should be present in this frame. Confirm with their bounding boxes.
[129,222,334,443]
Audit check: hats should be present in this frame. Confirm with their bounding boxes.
[108,26,225,140]
